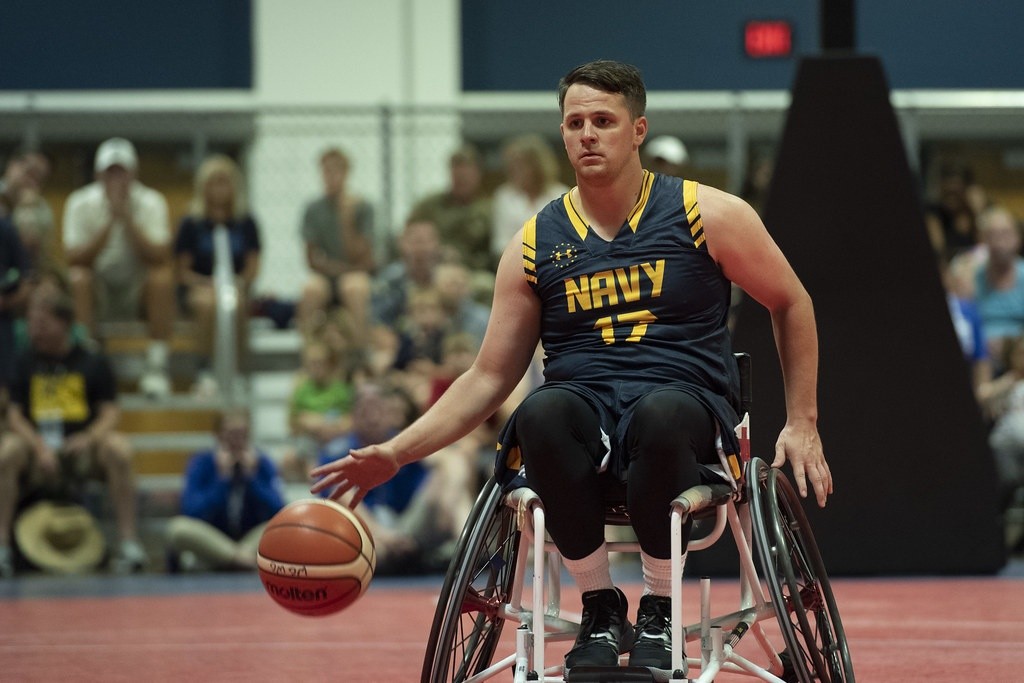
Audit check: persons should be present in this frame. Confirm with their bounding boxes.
[307,57,837,681]
[0,133,1024,568]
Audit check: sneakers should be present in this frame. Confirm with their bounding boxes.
[629,595,690,682]
[563,585,634,682]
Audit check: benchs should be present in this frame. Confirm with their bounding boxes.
[238,327,356,511]
[0,320,238,524]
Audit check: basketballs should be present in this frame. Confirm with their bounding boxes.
[257,497,378,617]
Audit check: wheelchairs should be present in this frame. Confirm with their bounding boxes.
[412,333,856,683]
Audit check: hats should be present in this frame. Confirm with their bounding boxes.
[644,134,688,166]
[94,137,138,174]
